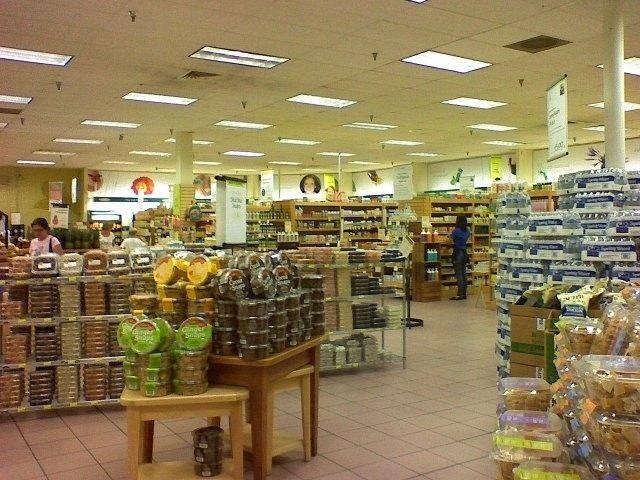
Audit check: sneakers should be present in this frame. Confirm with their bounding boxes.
[449,295,466,300]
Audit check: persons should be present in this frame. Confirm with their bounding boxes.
[450,213,469,300]
[119,227,148,250]
[99,222,116,252]
[303,178,317,194]
[26,219,66,255]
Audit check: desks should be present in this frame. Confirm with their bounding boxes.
[119,328,331,479]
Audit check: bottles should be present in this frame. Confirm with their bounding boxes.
[493,167,640,383]
[426,248,438,262]
[427,268,439,282]
